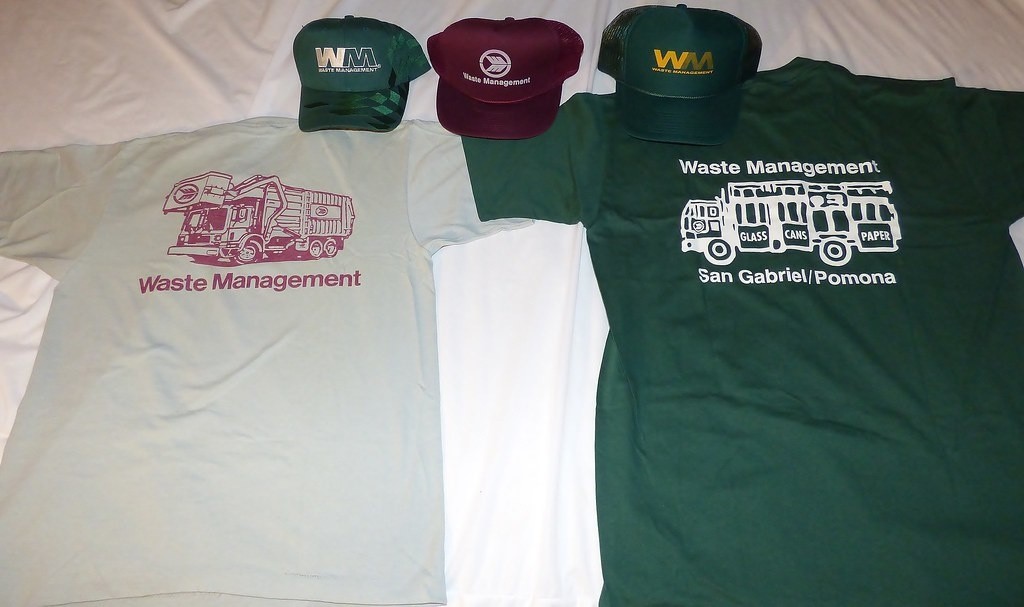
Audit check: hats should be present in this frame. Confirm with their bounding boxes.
[292,14,432,133]
[598,2,761,146]
[427,14,584,141]
